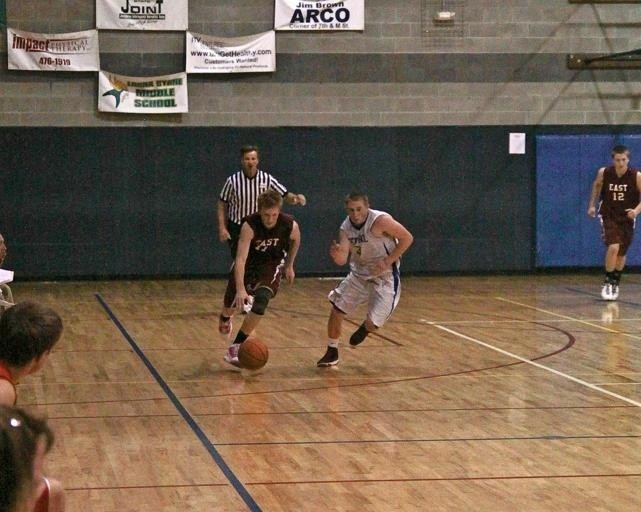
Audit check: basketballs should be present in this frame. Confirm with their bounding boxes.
[238,338,270,369]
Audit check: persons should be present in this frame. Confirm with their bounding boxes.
[218,189,304,365]
[317,190,416,368]
[220,142,309,257]
[587,145,641,301]
[0,231,67,512]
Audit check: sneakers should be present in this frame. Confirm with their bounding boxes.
[223,344,241,367]
[600,277,619,300]
[219,315,232,336]
[240,294,254,313]
[350,324,368,345]
[317,345,339,367]
